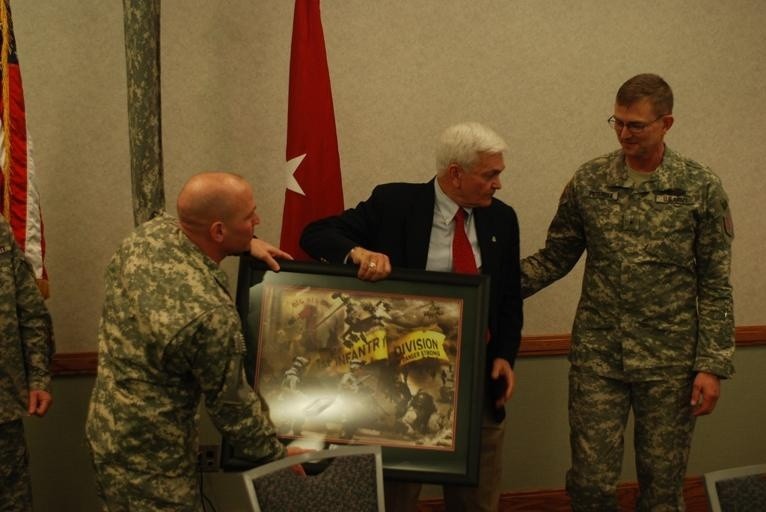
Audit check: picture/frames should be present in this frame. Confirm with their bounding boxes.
[218,257,492,488]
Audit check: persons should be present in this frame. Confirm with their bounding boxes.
[520,73,735,512]
[85,172,318,512]
[300,122,524,512]
[0,211,56,512]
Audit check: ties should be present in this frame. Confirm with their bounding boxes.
[451,210,477,274]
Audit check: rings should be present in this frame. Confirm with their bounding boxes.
[369,262,377,269]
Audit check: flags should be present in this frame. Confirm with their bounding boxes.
[0,0,49,300]
[280,0,345,263]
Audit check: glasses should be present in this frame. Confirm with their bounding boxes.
[606,114,663,134]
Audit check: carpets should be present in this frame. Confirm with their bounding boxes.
[414,459,764,512]
